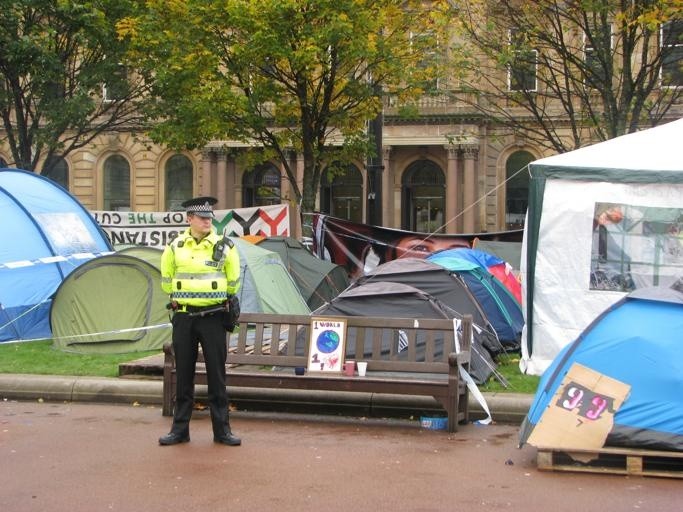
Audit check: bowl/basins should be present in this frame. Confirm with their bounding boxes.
[421,416,448,430]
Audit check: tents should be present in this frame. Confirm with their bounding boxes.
[321,256,503,360]
[424,245,524,302]
[271,281,500,391]
[45,242,175,352]
[516,118,682,378]
[423,252,525,352]
[210,235,309,330]
[241,234,355,311]
[0,166,114,347]
[514,286,682,451]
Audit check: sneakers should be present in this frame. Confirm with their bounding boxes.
[213,434,242,446]
[158,432,191,445]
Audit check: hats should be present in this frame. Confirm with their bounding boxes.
[181,197,218,219]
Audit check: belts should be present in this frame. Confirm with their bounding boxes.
[175,304,224,312]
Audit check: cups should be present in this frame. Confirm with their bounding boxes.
[342,361,368,376]
[295,367,306,375]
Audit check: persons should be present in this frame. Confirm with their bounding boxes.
[365,223,478,267]
[156,195,243,446]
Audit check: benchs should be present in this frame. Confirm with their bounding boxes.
[163,311,473,432]
